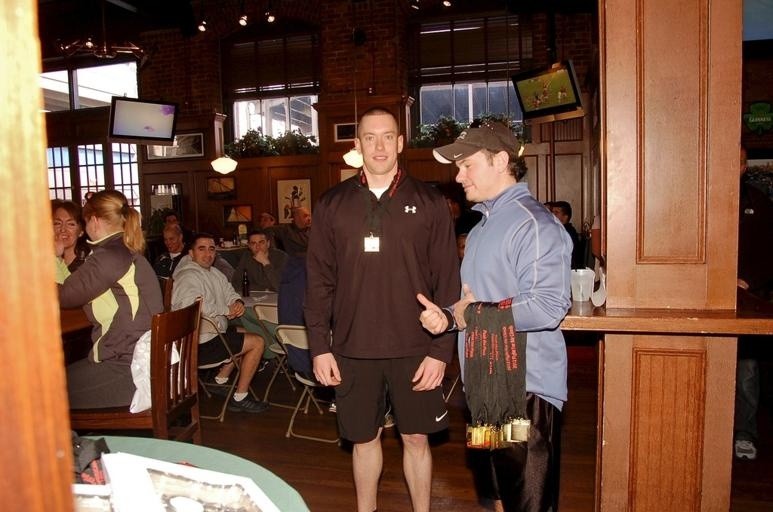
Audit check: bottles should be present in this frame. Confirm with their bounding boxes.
[219,238,223,248]
[242,270,250,297]
[232,232,235,245]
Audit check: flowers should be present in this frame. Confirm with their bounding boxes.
[432,119,459,146]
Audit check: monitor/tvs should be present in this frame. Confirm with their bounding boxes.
[511,59,583,118]
[107,96,178,145]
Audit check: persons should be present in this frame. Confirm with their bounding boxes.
[301,104,461,511]
[50,182,583,294]
[414,122,579,511]
[170,233,272,413]
[58,188,164,411]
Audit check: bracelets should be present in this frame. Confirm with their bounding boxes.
[440,308,455,333]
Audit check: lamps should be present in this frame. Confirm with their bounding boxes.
[211,81,238,174]
[52,0,147,61]
[198,0,275,32]
[342,73,363,167]
[411,0,420,9]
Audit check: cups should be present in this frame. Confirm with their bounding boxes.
[570,269,592,303]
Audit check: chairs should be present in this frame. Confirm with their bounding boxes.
[200,315,260,421]
[71,278,203,444]
[254,303,323,415]
[274,324,340,447]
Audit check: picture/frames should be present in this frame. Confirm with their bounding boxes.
[142,129,206,163]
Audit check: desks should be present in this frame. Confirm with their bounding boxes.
[234,290,279,309]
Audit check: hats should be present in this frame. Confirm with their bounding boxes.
[433,116,524,164]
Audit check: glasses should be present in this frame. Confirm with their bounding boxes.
[468,119,507,149]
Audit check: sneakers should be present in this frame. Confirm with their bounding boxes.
[734,440,756,461]
[206,378,234,397]
[228,398,271,413]
